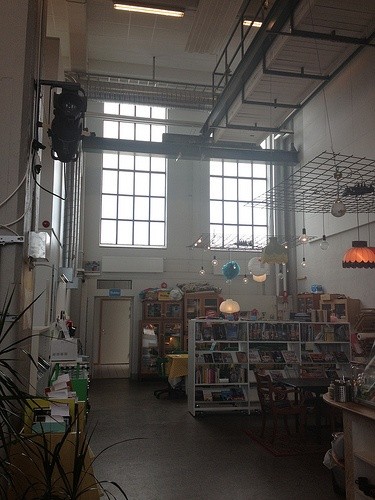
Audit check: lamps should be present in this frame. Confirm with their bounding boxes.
[242,16,264,28]
[331,165,346,218]
[340,186,375,270]
[0,432,100,500]
[49,337,79,362]
[260,42,289,265]
[112,1,186,18]
[298,191,330,267]
[219,249,268,314]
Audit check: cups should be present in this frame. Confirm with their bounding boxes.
[310,310,317,322]
[351,386,360,403]
[316,309,324,322]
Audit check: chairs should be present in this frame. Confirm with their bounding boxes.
[153,357,188,401]
[254,370,300,438]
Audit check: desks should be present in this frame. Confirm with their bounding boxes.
[166,354,189,390]
[277,377,341,443]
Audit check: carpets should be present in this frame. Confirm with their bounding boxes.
[242,425,329,458]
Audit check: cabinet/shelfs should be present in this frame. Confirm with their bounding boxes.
[322,391,375,500]
[138,295,221,381]
[296,292,361,328]
[183,316,352,417]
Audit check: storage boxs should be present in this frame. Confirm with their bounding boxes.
[50,368,89,409]
[328,449,345,499]
